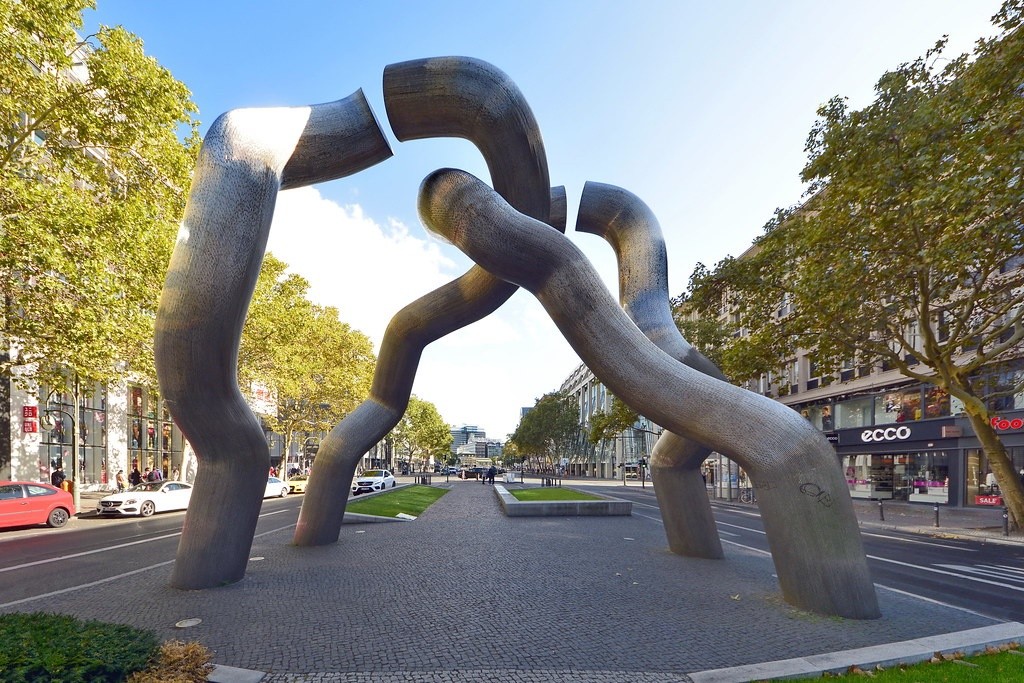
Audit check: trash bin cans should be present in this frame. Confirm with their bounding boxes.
[421,474,426,484]
[61,480,73,494]
[546,477,551,486]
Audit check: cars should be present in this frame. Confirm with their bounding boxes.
[351,469,396,496]
[263,477,290,498]
[287,476,310,494]
[449,467,457,474]
[96,480,193,518]
[0,480,76,528]
[440,468,450,476]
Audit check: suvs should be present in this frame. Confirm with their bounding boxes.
[458,468,489,479]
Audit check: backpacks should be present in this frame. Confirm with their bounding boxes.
[143,472,147,478]
[148,472,156,480]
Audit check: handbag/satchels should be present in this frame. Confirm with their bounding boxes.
[56,476,63,483]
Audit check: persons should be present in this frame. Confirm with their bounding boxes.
[115,467,180,490]
[802,410,811,422]
[269,463,308,480]
[51,466,66,489]
[537,469,539,475]
[820,408,832,431]
[482,465,496,484]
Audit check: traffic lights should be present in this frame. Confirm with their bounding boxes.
[442,455,446,460]
